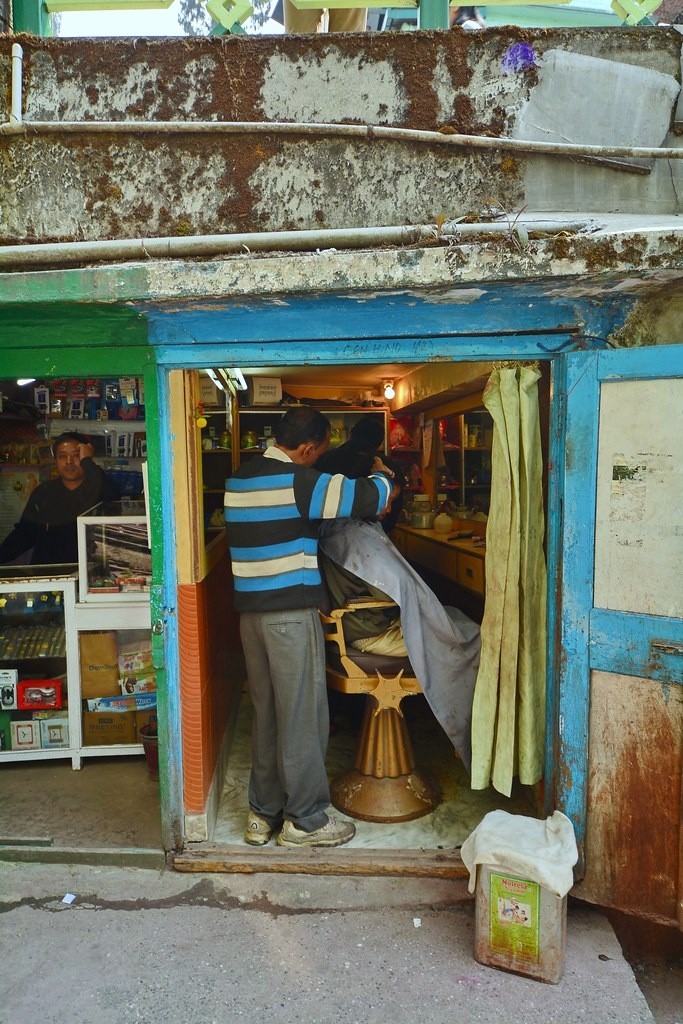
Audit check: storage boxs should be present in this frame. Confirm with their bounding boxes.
[248,375,282,407]
[0,670,69,752]
[199,378,223,407]
[79,633,157,746]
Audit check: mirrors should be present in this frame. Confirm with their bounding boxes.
[434,411,493,524]
[189,367,241,584]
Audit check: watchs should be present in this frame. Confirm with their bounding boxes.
[0,626,66,659]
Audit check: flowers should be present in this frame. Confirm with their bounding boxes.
[192,401,210,428]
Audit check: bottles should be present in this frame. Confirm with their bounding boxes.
[433,512,454,534]
[411,494,431,512]
[146,715,158,736]
[434,494,446,511]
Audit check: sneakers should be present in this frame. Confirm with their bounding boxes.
[245,810,273,846]
[277,818,356,848]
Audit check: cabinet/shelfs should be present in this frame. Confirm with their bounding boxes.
[237,406,486,600]
[201,407,231,533]
[437,409,493,514]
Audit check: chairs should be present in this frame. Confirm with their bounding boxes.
[316,596,441,824]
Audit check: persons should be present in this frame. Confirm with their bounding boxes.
[222,407,396,848]
[0,431,121,566]
[316,455,482,657]
[313,418,394,481]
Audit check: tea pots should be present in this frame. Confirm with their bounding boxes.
[402,503,437,529]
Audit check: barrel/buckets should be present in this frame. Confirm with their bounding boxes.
[139,723,159,782]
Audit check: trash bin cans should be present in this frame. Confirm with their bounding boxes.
[138,722,159,784]
